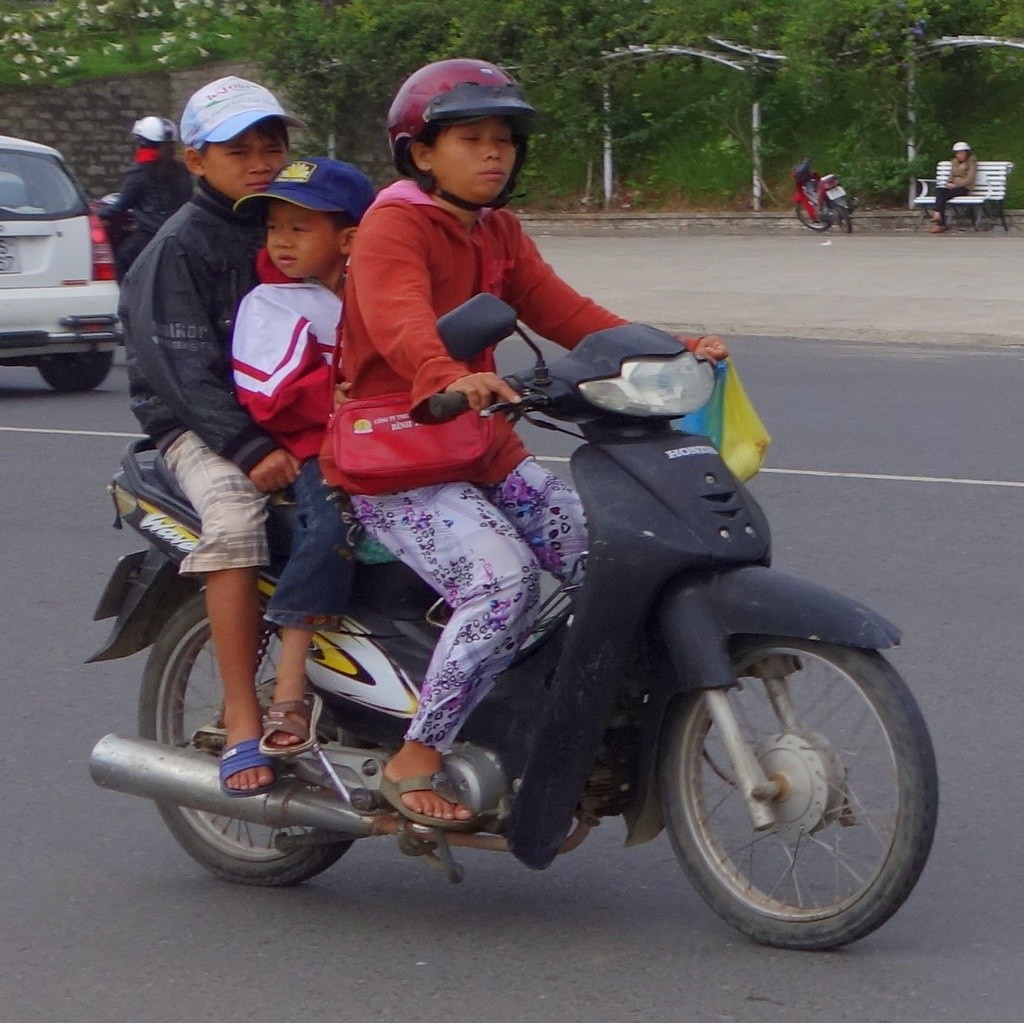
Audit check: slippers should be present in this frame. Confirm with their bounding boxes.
[218,737,277,800]
[259,692,324,757]
[378,768,473,830]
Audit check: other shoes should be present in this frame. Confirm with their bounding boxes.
[928,225,947,233]
[929,213,943,223]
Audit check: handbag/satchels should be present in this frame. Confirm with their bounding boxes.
[318,392,514,493]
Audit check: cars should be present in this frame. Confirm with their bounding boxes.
[0,136,123,391]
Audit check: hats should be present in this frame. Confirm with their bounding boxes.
[180,75,308,150]
[233,157,376,224]
[952,141,971,151]
[131,116,178,145]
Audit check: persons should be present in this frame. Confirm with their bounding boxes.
[233,158,379,755]
[116,76,305,799]
[335,58,730,829]
[93,117,196,286]
[928,141,978,233]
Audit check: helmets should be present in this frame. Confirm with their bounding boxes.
[386,58,536,212]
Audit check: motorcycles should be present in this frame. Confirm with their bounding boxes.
[93,190,136,247]
[792,156,859,233]
[80,286,944,957]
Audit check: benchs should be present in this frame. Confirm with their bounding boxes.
[913,161,1013,232]
